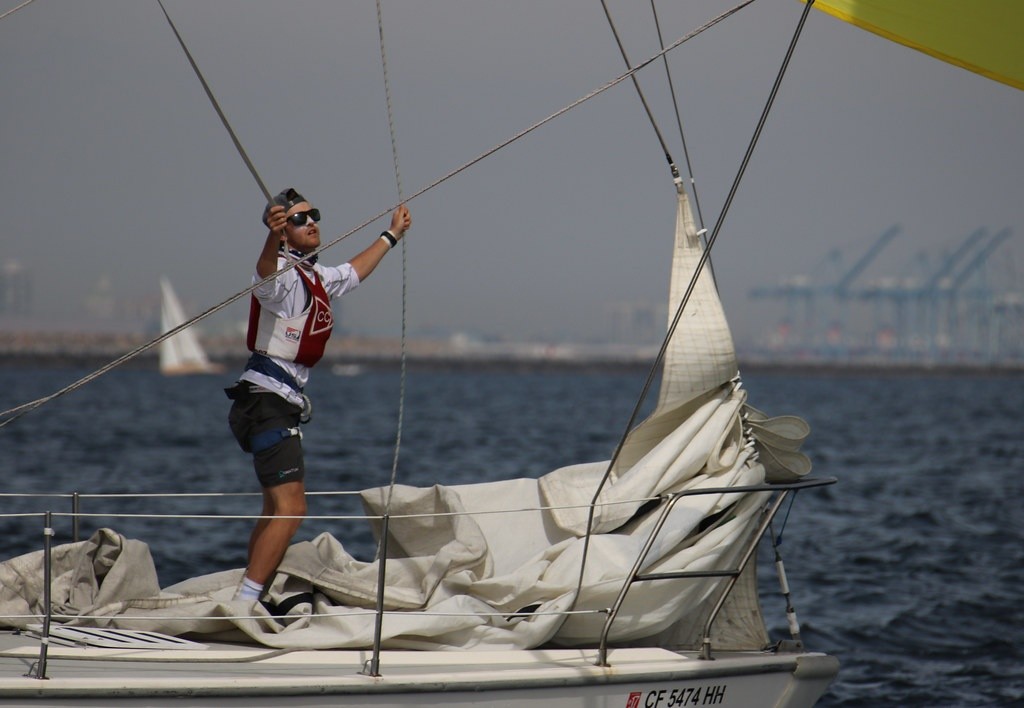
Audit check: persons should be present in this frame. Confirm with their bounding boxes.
[224,188,412,600]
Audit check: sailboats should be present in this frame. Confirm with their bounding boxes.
[0,1,1023,708]
[159,276,227,375]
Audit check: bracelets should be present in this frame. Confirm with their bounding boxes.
[380,230,398,248]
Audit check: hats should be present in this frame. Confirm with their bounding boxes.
[262,187,305,229]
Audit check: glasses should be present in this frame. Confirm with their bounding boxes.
[288,208,321,227]
